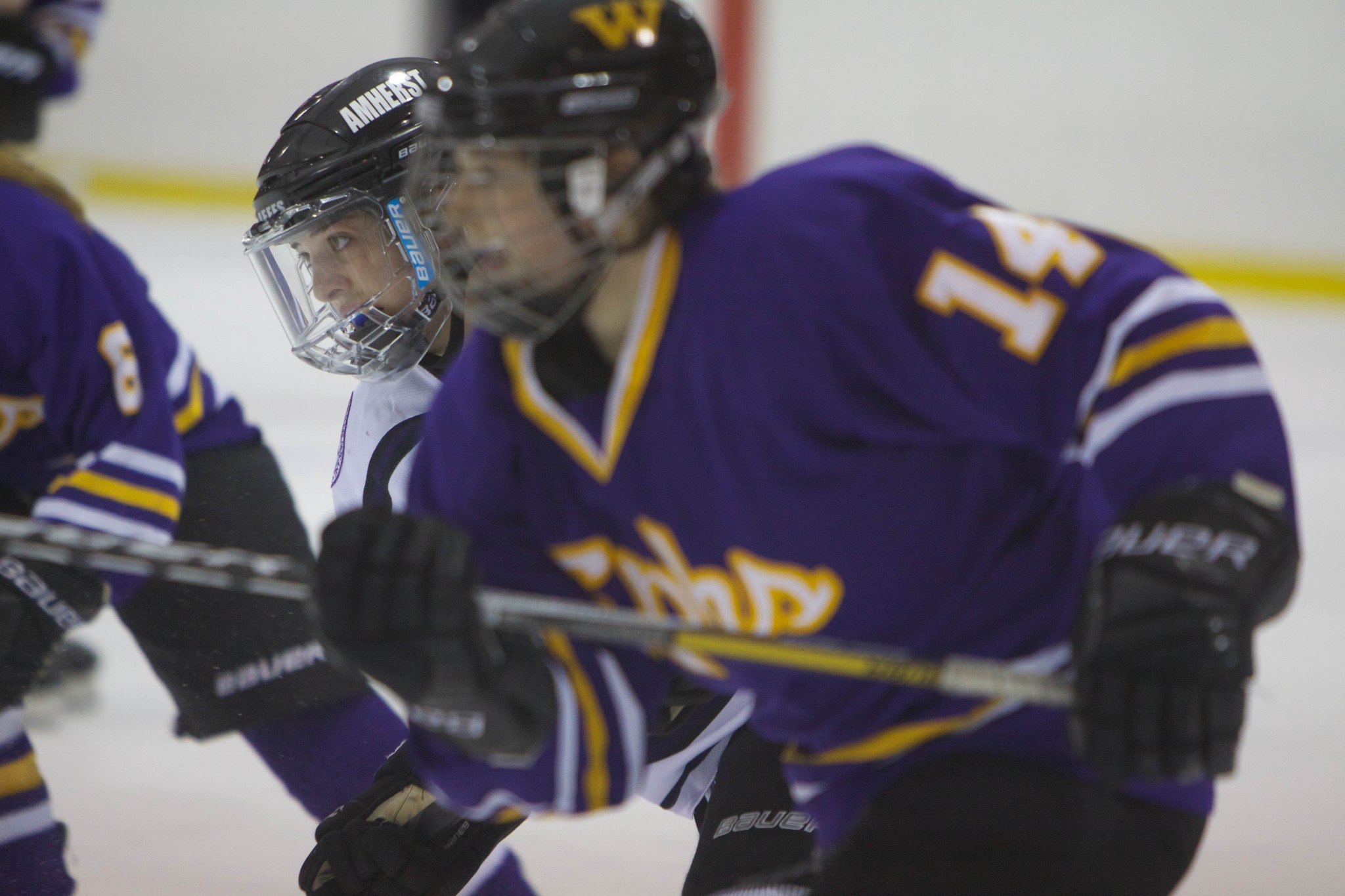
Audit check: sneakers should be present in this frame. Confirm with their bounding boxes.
[21,643,99,713]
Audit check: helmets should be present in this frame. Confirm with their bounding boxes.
[0,0,106,146]
[443,0,721,259]
[242,57,452,255]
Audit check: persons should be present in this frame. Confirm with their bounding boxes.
[0,0,813,896]
[309,0,1299,896]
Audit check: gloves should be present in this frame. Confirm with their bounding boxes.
[1066,474,1289,788]
[307,507,553,760]
[298,734,530,896]
[0,551,107,709]
